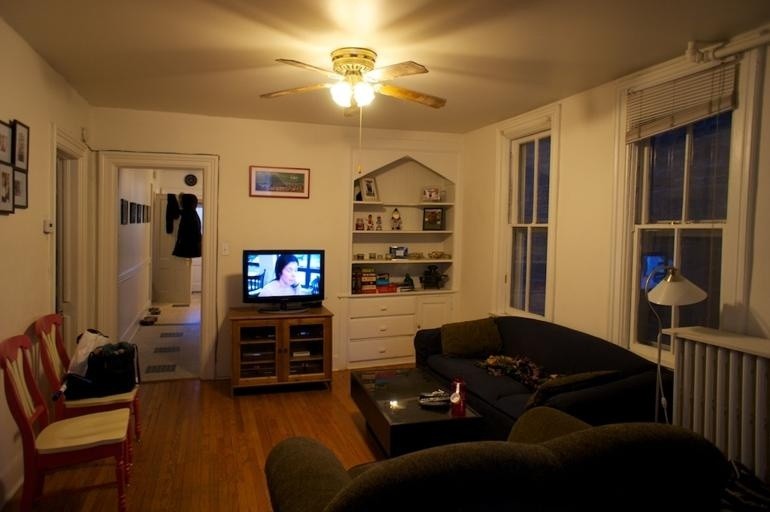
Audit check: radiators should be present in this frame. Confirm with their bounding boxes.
[662,324,770,486]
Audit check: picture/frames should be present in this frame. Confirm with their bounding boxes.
[12,169,29,210]
[12,119,31,172]
[0,120,11,165]
[248,165,311,199]
[0,164,15,217]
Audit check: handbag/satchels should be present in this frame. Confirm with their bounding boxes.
[85,338,140,394]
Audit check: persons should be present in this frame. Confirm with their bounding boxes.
[258,254,303,297]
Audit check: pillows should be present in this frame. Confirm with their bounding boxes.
[442,317,501,357]
[522,370,623,410]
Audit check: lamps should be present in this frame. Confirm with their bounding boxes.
[644,264,707,426]
[330,46,377,174]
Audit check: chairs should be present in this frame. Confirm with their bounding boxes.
[33,315,142,465]
[1,335,132,512]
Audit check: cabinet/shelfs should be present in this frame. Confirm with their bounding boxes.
[228,305,334,398]
[338,153,460,371]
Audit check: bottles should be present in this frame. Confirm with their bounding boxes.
[449,376,466,419]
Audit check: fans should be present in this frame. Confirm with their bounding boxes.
[259,46,446,110]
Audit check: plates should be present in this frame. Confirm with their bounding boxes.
[418,397,449,406]
[421,392,450,398]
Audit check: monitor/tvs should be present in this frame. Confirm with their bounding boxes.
[241,249,326,314]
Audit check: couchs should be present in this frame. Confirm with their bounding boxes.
[413,314,674,441]
[265,407,730,512]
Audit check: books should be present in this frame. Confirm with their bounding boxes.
[354,267,378,294]
[376,273,397,293]
